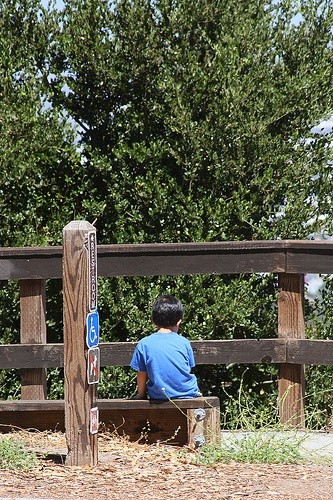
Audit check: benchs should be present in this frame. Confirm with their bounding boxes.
[1,394,221,453]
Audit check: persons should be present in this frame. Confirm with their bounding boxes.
[130,295,204,399]
